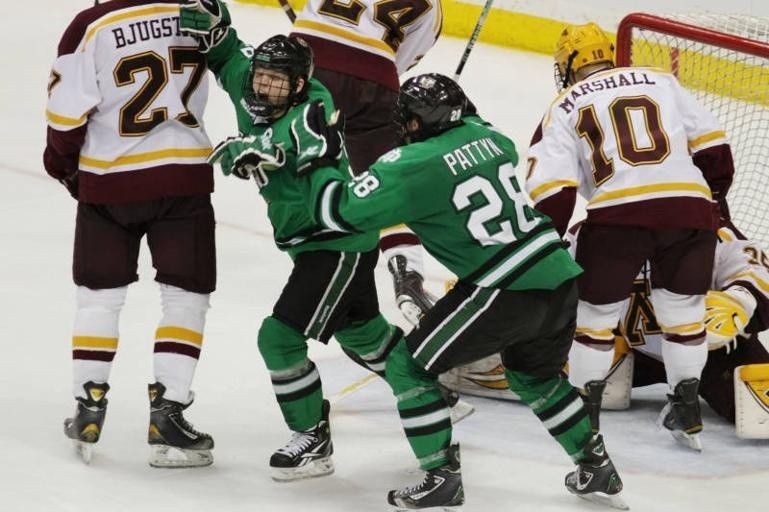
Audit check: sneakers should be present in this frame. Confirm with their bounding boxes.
[63,381,110,442]
[270,399,333,467]
[388,443,464,508]
[565,434,623,494]
[663,378,703,433]
[149,382,214,449]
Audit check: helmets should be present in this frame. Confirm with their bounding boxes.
[393,73,465,140]
[553,18,614,92]
[241,35,314,116]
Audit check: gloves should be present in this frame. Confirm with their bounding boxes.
[288,99,343,175]
[179,0,231,54]
[207,137,262,175]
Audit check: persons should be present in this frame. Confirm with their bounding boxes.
[439,221,769,440]
[290,0,443,329]
[287,72,621,506]
[517,22,735,453]
[43,1,213,451]
[175,0,458,470]
